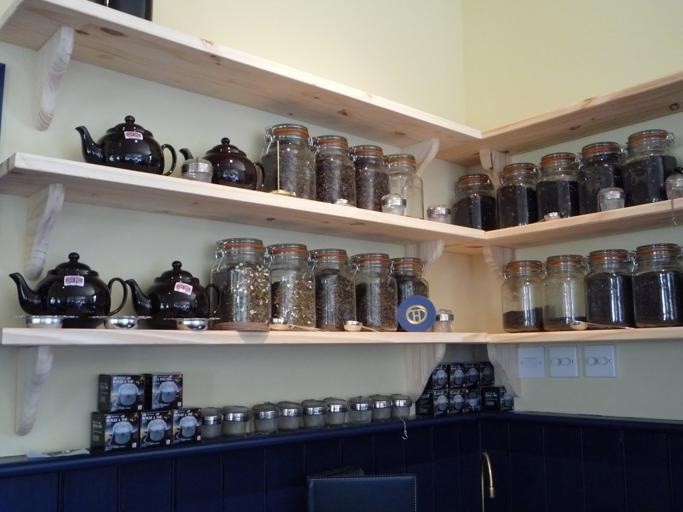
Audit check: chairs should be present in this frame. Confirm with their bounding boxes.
[304,471,420,511]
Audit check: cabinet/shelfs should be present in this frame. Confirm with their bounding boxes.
[0,0,482,344]
[482,68,682,343]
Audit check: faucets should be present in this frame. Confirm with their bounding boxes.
[477,450,496,512]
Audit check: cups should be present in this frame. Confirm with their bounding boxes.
[15,313,80,328]
[87,315,154,329]
[161,317,221,330]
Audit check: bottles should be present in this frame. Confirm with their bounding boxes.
[453,126,679,230]
[260,122,451,224]
[207,236,431,332]
[499,242,683,330]
[200,391,414,441]
[432,308,457,333]
[665,171,683,199]
[180,156,215,183]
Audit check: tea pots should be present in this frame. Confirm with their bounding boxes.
[180,135,265,191]
[8,252,129,327]
[75,114,176,177]
[124,259,223,328]
